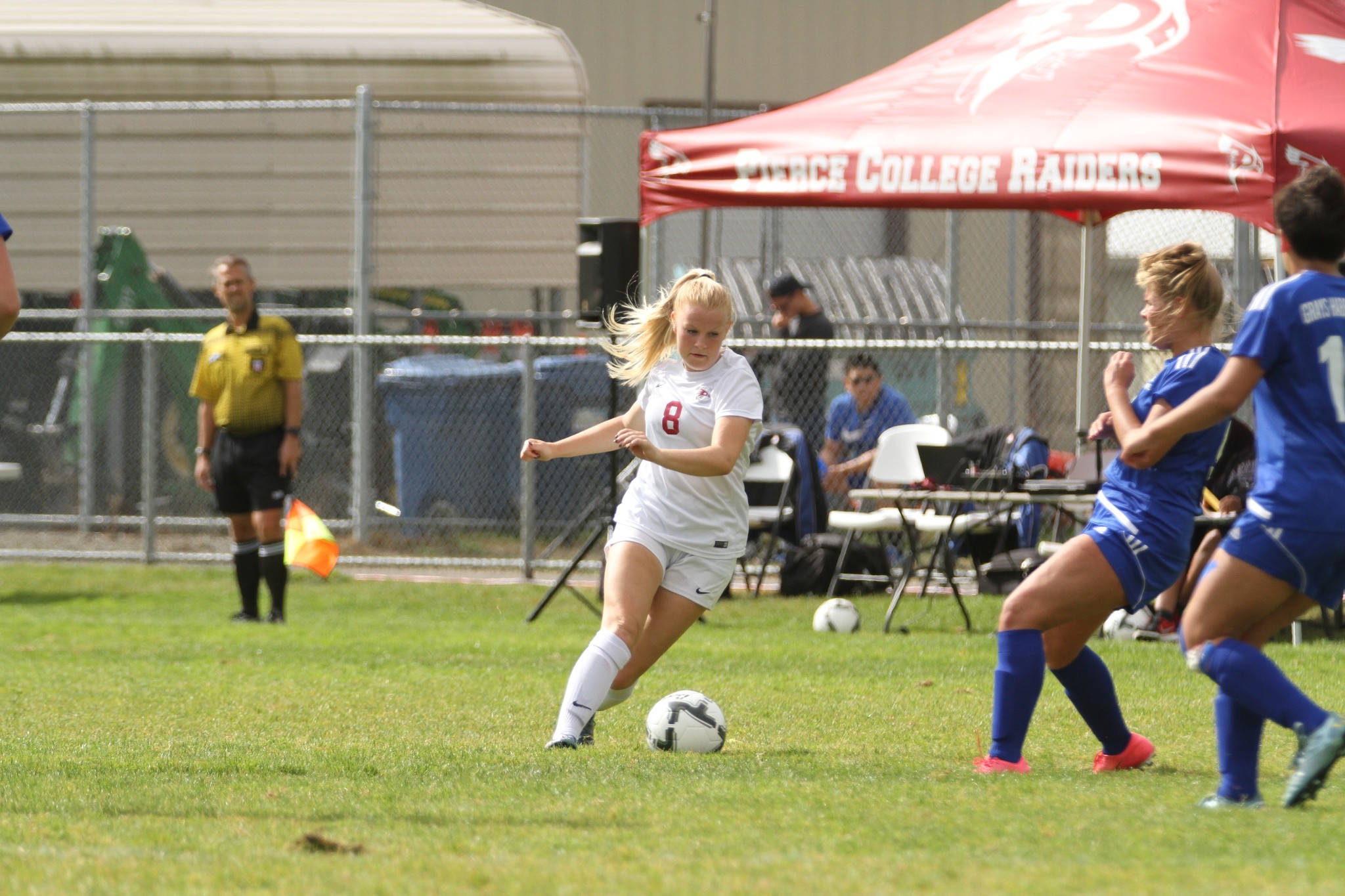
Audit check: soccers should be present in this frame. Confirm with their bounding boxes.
[1102,604,1153,641]
[645,690,726,755]
[812,597,861,633]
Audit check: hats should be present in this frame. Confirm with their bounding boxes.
[769,275,810,296]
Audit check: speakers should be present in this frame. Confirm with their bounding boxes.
[578,215,638,317]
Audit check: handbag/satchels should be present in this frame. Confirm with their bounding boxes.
[779,533,893,597]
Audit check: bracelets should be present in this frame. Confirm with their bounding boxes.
[194,446,211,455]
[284,426,301,435]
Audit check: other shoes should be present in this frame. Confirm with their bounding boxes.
[230,612,258,620]
[263,611,284,622]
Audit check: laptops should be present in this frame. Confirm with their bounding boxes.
[918,444,1002,494]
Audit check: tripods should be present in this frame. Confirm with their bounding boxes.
[526,321,642,623]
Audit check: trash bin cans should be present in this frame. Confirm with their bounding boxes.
[373,353,614,528]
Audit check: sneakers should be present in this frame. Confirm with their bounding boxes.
[577,717,597,745]
[1134,608,1179,641]
[546,735,578,749]
[1282,711,1345,807]
[974,730,1031,774]
[1091,731,1156,774]
[1196,794,1263,809]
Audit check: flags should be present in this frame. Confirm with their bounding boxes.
[283,498,340,581]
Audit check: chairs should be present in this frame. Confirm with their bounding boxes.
[525,424,1049,621]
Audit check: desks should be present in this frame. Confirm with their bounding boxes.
[849,490,1097,632]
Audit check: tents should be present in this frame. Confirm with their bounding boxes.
[638,0,1345,456]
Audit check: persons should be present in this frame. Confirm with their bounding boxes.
[187,255,306,623]
[0,213,20,342]
[1123,164,1345,809]
[970,243,1231,774]
[749,271,836,451]
[519,269,763,745]
[818,351,917,575]
[1134,417,1256,641]
